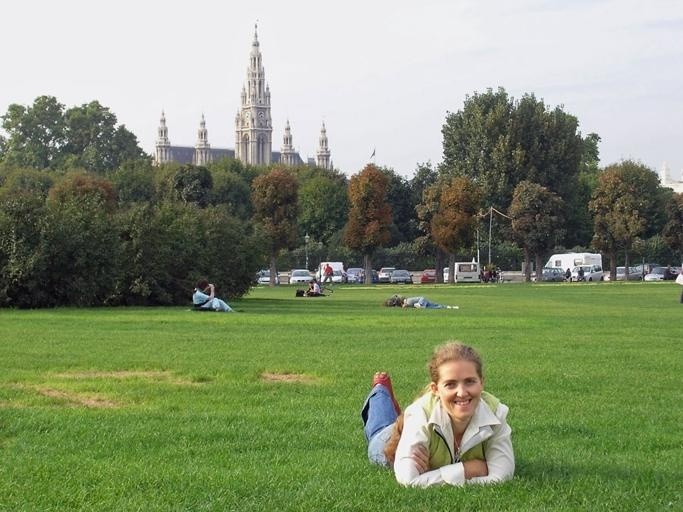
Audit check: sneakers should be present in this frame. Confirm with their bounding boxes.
[373,371,401,417]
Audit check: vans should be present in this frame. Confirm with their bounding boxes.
[454,261,482,283]
[544,252,602,267]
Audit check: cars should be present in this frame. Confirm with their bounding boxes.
[608,261,683,281]
[421,268,437,284]
[443,267,450,283]
[257,268,280,286]
[541,265,605,282]
[347,267,414,284]
[287,269,314,285]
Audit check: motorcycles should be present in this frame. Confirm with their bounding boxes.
[484,268,505,285]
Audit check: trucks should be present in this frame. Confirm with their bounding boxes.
[318,261,345,283]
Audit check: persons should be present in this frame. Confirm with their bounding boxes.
[303,263,335,297]
[192,278,236,312]
[563,266,587,282]
[663,264,677,280]
[360,341,515,490]
[480,266,500,283]
[399,296,460,311]
